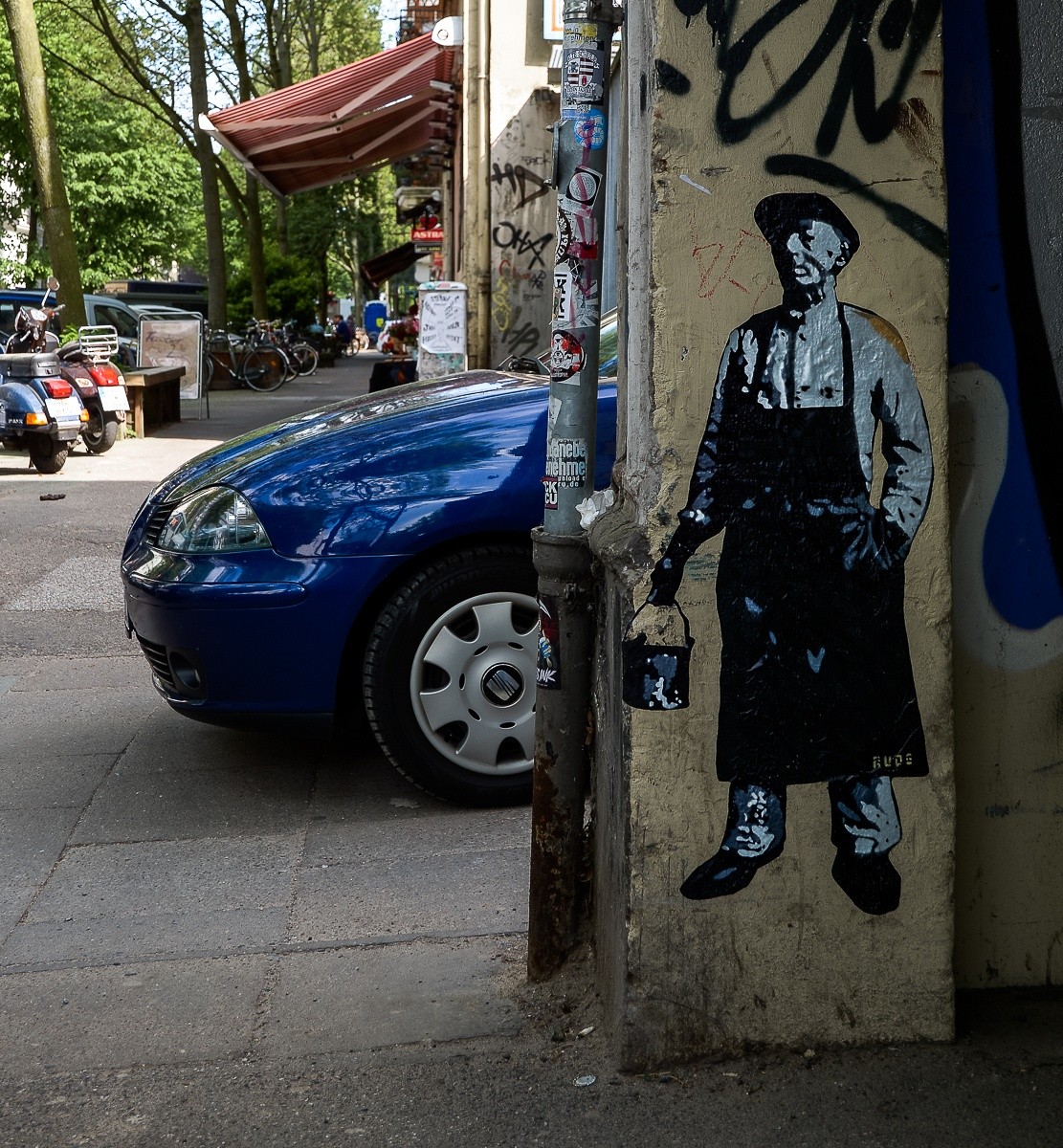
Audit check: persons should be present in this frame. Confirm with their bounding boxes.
[405,305,418,323]
[334,315,355,343]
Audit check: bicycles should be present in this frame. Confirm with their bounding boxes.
[242,312,361,376]
[202,324,289,394]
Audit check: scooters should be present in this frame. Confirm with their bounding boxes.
[0,276,132,454]
[0,342,92,475]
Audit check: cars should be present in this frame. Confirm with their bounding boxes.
[0,289,244,390]
[118,305,619,810]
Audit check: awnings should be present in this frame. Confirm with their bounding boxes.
[359,241,428,295]
[198,31,455,199]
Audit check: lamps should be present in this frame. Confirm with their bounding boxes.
[423,80,463,174]
